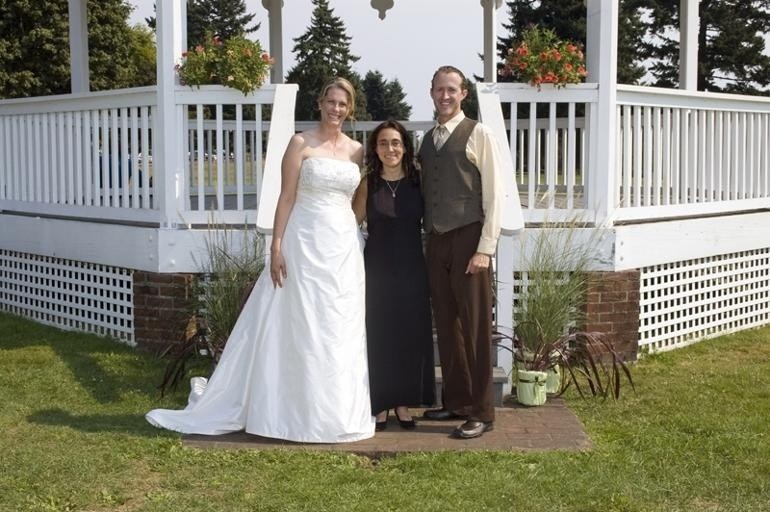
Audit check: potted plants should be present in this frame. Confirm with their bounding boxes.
[487,200,638,410]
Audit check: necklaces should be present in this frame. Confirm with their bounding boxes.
[330,133,339,156]
[385,173,402,198]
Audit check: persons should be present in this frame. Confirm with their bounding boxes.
[140,74,377,445]
[352,118,439,434]
[416,65,507,439]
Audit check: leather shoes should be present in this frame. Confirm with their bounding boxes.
[423,406,471,421]
[394,405,415,430]
[455,416,495,438]
[376,408,389,431]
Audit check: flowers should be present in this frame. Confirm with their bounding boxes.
[177,27,275,98]
[499,24,590,94]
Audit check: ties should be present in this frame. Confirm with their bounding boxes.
[434,127,448,152]
